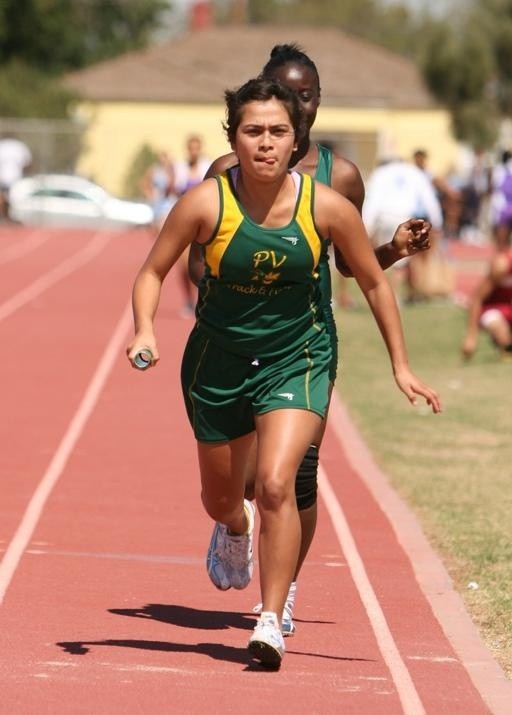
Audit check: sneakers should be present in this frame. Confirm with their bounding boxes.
[279,581,297,636]
[247,610,284,666]
[206,499,255,591]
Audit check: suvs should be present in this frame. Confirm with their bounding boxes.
[6,175,156,231]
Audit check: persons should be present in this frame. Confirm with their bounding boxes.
[188,43,432,635]
[126,74,442,669]
[338,142,512,359]
[136,151,175,234]
[165,136,212,318]
